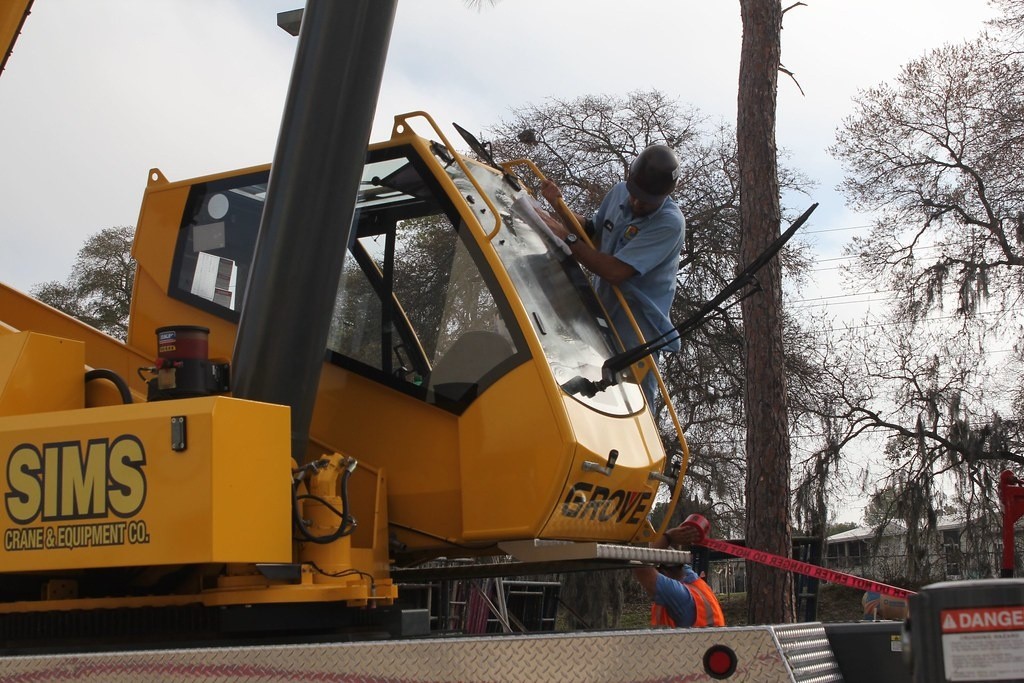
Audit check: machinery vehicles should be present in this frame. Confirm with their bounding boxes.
[1,2,822,639]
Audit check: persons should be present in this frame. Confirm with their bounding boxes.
[534,146,686,417]
[634,526,726,629]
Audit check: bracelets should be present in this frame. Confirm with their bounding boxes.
[663,533,672,545]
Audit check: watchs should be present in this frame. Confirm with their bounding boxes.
[565,234,578,243]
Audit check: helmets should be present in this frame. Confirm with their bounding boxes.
[626,146,679,200]
